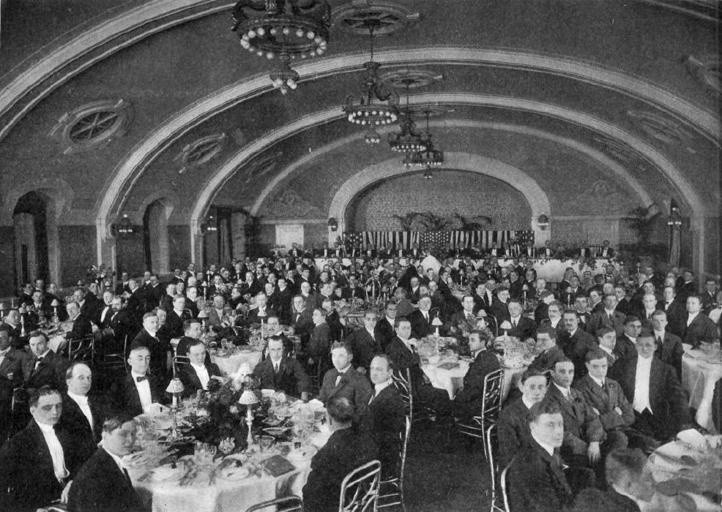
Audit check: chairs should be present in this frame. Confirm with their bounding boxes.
[309,345,330,392]
[339,460,381,512]
[379,416,410,510]
[392,367,453,457]
[486,423,519,512]
[246,495,304,512]
[47,320,129,372]
[458,368,507,462]
[173,356,191,379]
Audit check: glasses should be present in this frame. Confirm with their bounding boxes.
[36,400,64,412]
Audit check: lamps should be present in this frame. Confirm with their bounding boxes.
[233,1,444,179]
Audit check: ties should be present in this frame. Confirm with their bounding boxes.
[655,334,665,361]
[273,362,280,374]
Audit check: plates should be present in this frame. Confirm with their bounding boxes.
[119,396,327,483]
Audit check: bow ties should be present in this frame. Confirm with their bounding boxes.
[335,371,344,377]
[136,375,150,382]
[36,357,44,363]
[297,311,302,316]
